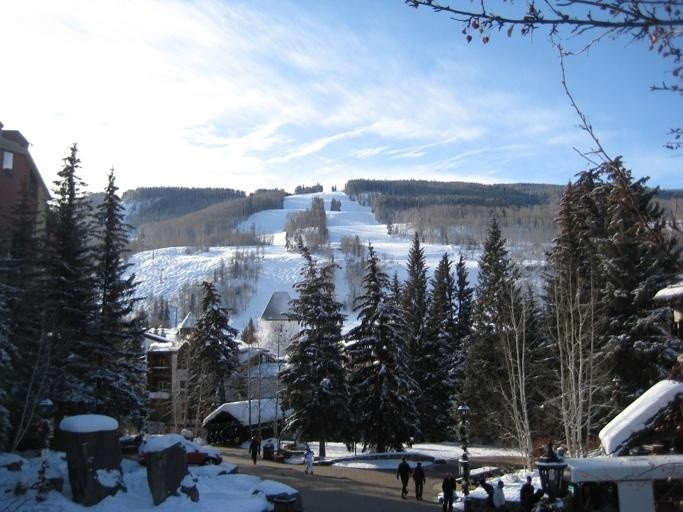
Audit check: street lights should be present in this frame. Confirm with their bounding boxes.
[534,443,567,512]
[457,401,471,495]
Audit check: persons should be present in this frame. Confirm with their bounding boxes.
[481,478,493,506]
[395,456,411,498]
[247,431,260,465]
[491,480,508,512]
[519,475,534,512]
[441,471,456,511]
[412,462,424,501]
[304,444,316,475]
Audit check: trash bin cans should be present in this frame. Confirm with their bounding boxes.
[182,430,194,441]
[60,415,123,507]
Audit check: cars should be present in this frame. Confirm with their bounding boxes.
[274,441,314,459]
[119,436,222,465]
